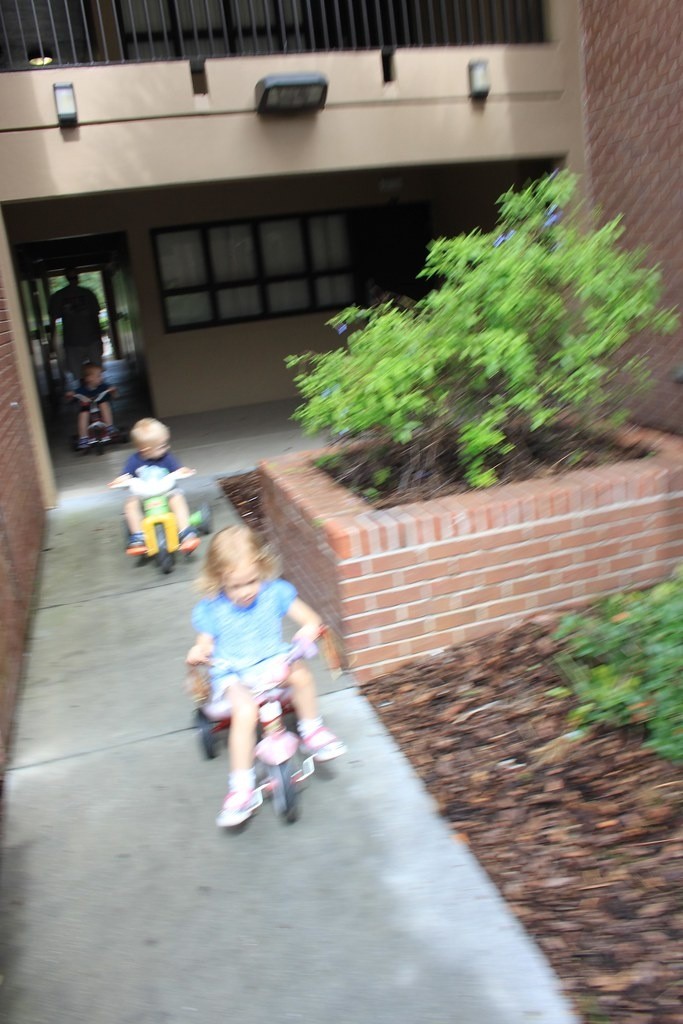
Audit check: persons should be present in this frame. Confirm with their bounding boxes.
[110,423,201,555]
[48,267,104,380]
[186,527,348,828]
[65,362,119,449]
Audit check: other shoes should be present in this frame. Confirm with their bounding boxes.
[218,787,261,827]
[300,725,347,762]
[125,533,147,556]
[78,437,89,449]
[178,528,199,552]
[105,426,117,438]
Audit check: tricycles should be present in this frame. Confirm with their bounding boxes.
[70,386,129,456]
[109,467,213,576]
[188,622,335,829]
